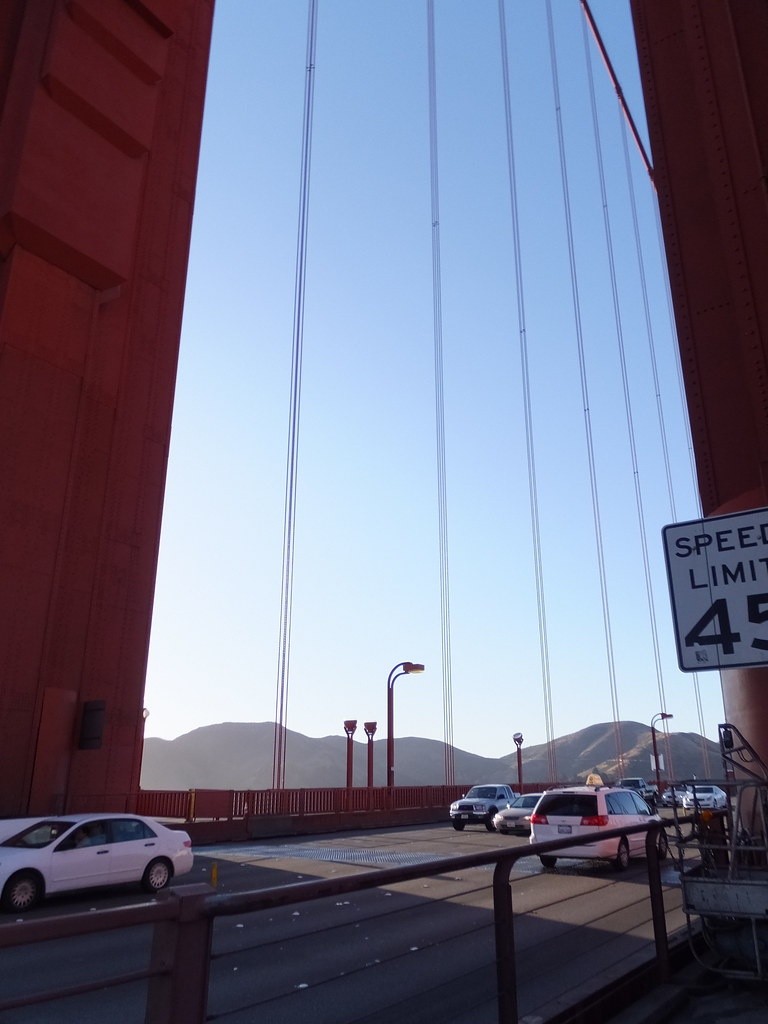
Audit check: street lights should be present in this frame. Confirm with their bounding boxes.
[651,713,673,787]
[512,733,523,793]
[364,722,377,787]
[387,662,424,786]
[343,720,357,788]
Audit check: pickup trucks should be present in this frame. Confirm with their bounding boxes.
[449,784,522,832]
[617,778,659,806]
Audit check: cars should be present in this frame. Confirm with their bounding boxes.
[661,783,690,807]
[493,793,543,834]
[683,786,727,810]
[0,813,194,912]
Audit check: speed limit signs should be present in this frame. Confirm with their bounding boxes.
[662,508,768,673]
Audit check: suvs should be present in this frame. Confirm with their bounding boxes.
[529,784,668,868]
